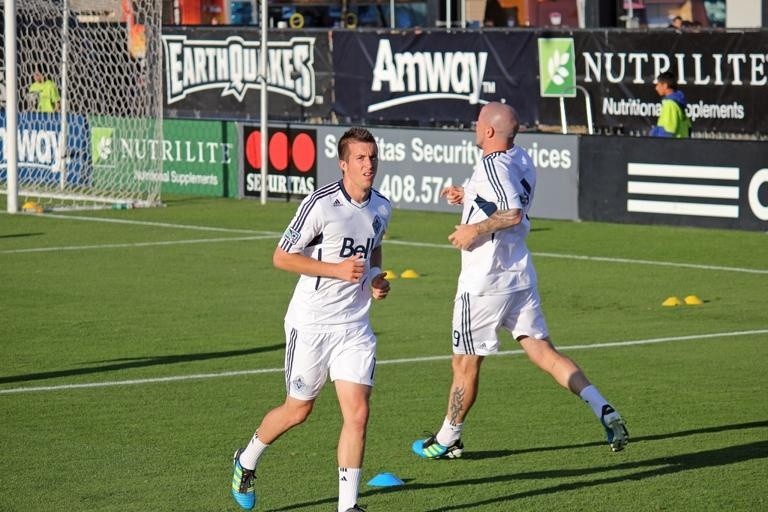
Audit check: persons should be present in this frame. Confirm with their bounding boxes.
[28,68,60,114]
[645,71,694,140]
[664,13,685,35]
[411,102,628,463]
[228,125,393,512]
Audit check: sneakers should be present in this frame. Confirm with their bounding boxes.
[411,434,465,460]
[230,446,257,510]
[599,403,629,452]
[336,504,366,512]
